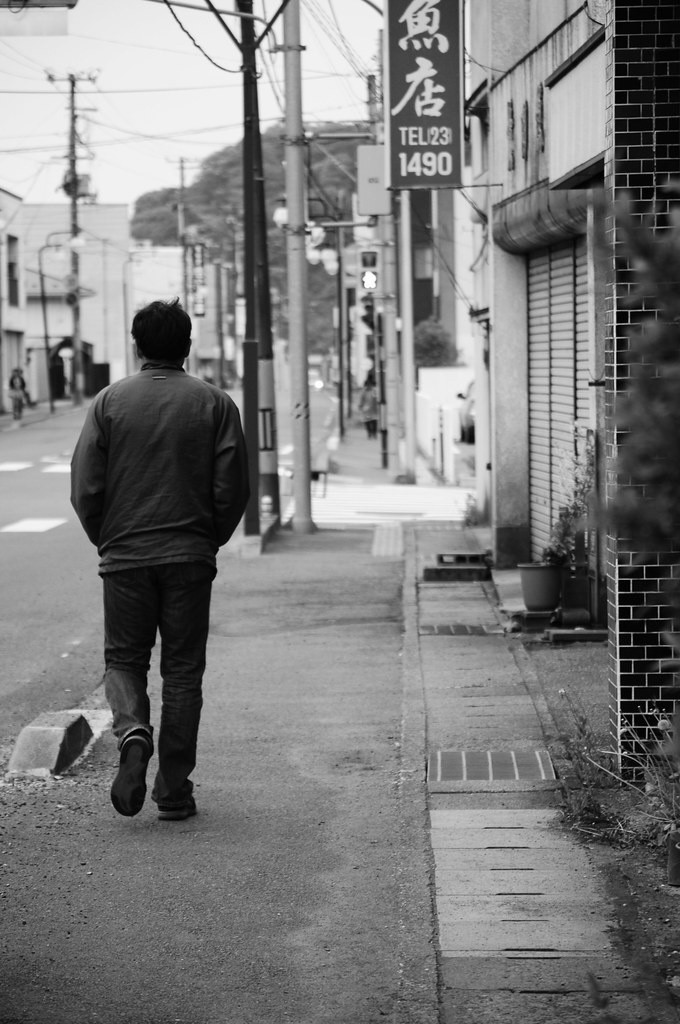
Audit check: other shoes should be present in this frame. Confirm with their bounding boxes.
[110,736,150,817]
[157,798,197,819]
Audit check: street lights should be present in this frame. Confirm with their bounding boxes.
[36,236,87,414]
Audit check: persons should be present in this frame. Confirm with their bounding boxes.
[70,296,250,821]
[359,378,377,440]
[10,368,26,412]
